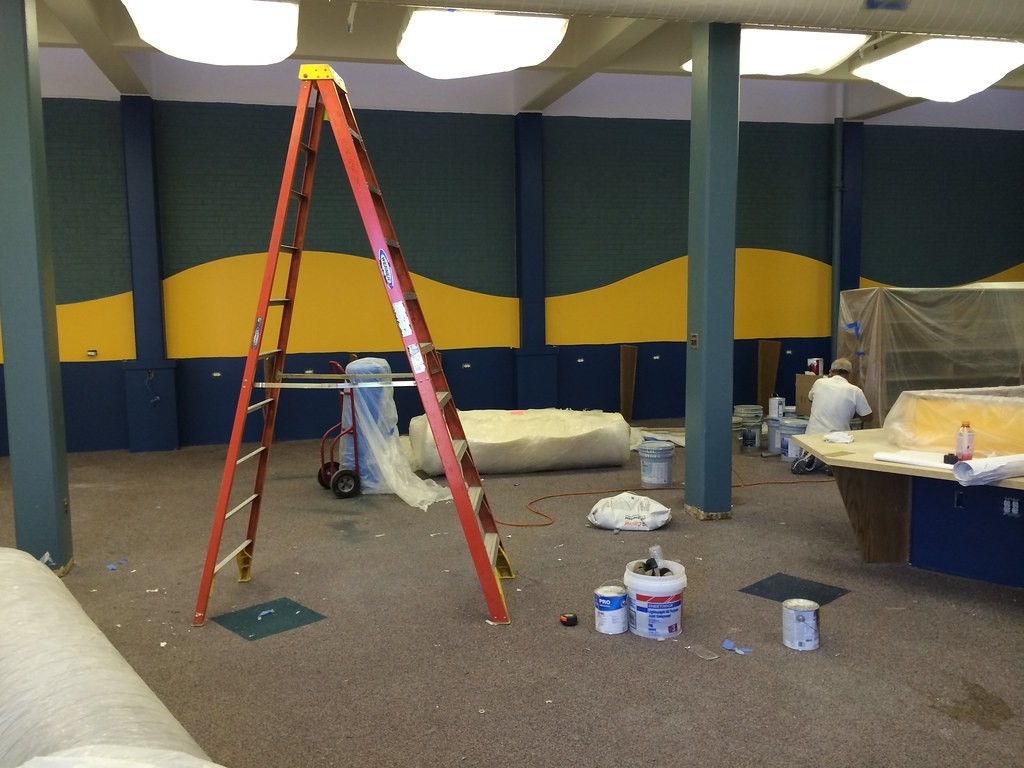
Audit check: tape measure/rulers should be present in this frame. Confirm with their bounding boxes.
[560,612,578,627]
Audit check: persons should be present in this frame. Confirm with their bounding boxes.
[790,358,874,477]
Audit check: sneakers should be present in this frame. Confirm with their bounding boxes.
[790,448,815,474]
[823,462,832,476]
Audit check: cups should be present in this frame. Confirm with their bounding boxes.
[805,371,809,375]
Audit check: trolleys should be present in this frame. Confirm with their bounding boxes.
[318,359,362,499]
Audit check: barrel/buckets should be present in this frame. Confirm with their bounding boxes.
[593,579,628,635]
[781,598,820,651]
[638,440,676,488]
[808,357,823,377]
[624,559,687,640]
[732,392,863,475]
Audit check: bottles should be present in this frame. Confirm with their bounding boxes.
[955,420,974,460]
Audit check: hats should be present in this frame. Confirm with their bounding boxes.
[830,357,853,373]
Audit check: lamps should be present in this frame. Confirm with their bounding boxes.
[395,4,570,80]
[847,37,1024,104]
[121,0,302,70]
[680,26,871,75]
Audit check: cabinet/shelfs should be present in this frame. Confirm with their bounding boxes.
[839,289,1024,428]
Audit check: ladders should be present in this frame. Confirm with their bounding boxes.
[192,63,516,627]
